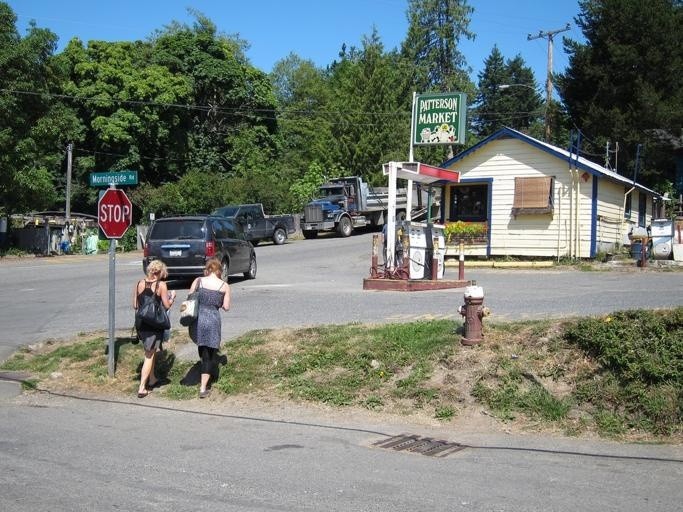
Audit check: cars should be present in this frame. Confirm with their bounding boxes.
[143,215,257,285]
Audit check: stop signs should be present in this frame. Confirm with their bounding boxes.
[97,188,133,239]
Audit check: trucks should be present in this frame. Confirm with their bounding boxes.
[300,176,407,237]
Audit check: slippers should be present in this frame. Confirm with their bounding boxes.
[138,389,152,398]
[149,376,160,387]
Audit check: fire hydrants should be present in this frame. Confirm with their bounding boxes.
[458,286,491,344]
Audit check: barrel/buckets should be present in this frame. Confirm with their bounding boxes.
[630,243,651,260]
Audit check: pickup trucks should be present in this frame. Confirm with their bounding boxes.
[213,204,295,245]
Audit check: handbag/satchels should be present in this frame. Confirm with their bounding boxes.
[137,282,171,329]
[179,278,200,324]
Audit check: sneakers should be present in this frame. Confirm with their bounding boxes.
[198,390,210,398]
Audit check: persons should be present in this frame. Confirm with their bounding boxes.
[131,260,175,398]
[179,259,231,398]
